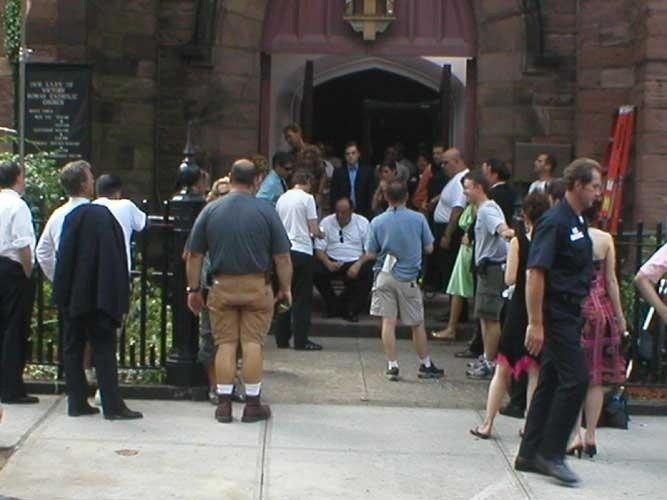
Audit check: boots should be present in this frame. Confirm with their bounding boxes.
[242,396,271,422]
[215,393,232,422]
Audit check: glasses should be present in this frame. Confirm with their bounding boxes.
[339,230,343,242]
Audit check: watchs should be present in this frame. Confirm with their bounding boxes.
[187,286,200,294]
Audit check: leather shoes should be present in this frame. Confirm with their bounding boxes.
[69,407,100,416]
[105,409,142,419]
[515,452,578,482]
[2,395,38,404]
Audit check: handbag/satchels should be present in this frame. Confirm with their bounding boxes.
[582,390,629,430]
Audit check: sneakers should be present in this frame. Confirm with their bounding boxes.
[295,342,322,350]
[431,326,497,380]
[386,367,399,381]
[418,361,444,378]
[499,403,524,418]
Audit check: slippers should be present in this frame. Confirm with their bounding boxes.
[470,426,489,439]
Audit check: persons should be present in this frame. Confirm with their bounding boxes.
[32,160,145,420]
[201,124,521,379]
[186,158,293,423]
[0,160,40,405]
[90,173,149,280]
[469,154,628,485]
[632,241,667,324]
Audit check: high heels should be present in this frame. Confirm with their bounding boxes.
[565,444,583,458]
[586,444,596,457]
